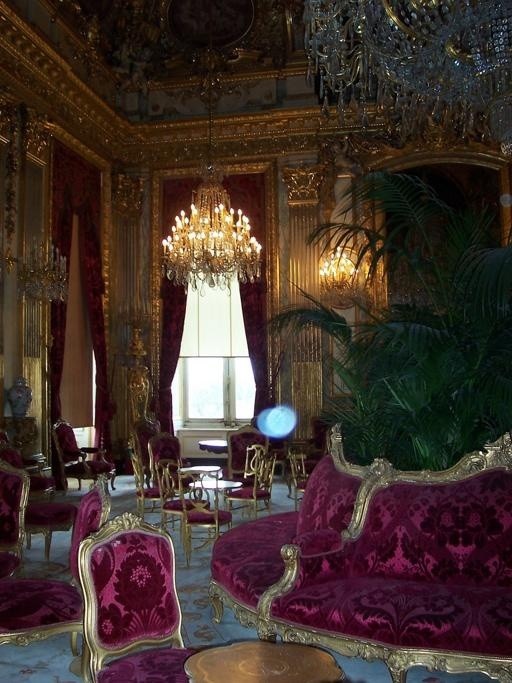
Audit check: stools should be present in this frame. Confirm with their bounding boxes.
[21,499,80,561]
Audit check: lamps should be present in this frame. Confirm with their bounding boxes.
[157,17,264,297]
[317,244,356,287]
[17,240,68,304]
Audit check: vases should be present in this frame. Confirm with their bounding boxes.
[7,375,32,417]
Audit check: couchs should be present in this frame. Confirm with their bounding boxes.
[209,420,511,683]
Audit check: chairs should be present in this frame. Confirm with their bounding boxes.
[122,405,331,574]
[0,452,35,587]
[0,471,113,683]
[50,414,117,496]
[1,433,55,503]
[73,511,200,682]
